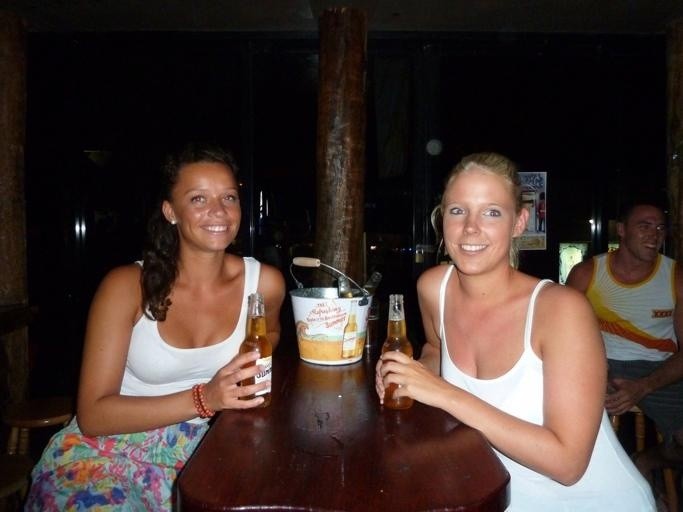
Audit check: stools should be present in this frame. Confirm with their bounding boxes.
[607,401,680,510]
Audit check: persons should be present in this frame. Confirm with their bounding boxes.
[374,153,655,512]
[565,204,683,504]
[24,146,287,512]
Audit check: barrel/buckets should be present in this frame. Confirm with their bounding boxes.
[288,256,373,366]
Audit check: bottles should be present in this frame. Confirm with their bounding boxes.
[378,292,416,411]
[336,273,354,299]
[339,298,360,361]
[236,291,275,409]
[359,270,383,297]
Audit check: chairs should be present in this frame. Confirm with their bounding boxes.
[0,304,77,456]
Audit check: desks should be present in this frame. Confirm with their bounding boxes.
[176,328,510,507]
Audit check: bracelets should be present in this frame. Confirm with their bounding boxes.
[192,384,216,418]
